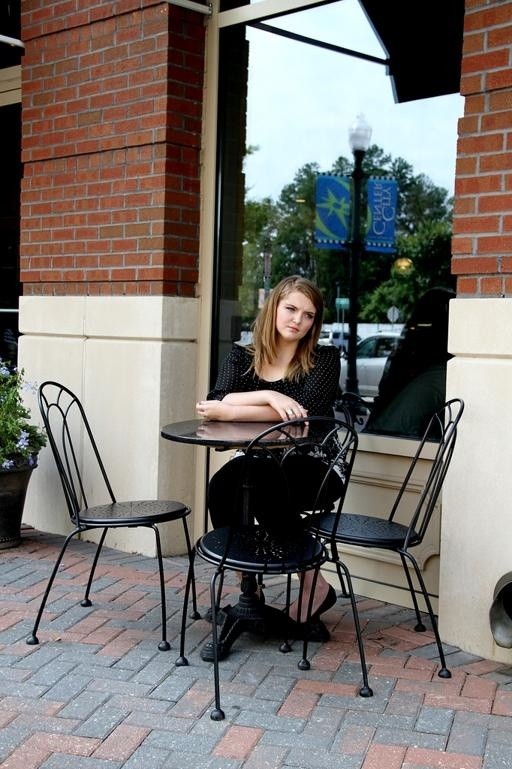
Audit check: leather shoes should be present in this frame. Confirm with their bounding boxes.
[278,579,341,620]
[257,584,266,605]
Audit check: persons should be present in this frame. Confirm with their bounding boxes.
[195,275,340,629]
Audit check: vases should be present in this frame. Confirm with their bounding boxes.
[0,470,32,549]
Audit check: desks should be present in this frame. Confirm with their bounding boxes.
[159,420,329,667]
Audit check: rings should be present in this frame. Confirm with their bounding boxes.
[286,409,293,415]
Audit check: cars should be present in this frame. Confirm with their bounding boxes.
[340,333,406,397]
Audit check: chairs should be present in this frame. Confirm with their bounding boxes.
[343,393,380,433]
[222,404,353,589]
[176,420,371,721]
[299,399,464,694]
[25,381,203,651]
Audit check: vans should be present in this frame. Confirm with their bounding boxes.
[318,328,362,352]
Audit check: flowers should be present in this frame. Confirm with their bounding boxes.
[0,359,46,470]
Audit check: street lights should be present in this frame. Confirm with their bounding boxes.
[262,223,279,309]
[343,109,372,415]
